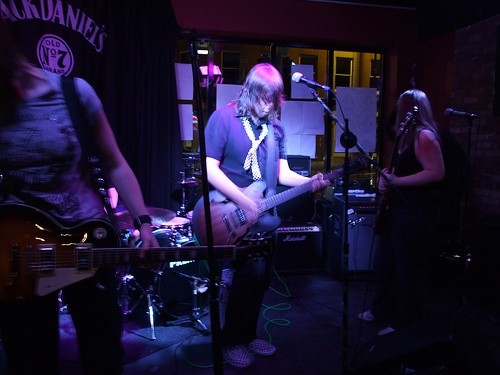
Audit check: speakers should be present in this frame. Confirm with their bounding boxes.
[272,228,323,276]
[346,317,461,375]
[344,206,379,272]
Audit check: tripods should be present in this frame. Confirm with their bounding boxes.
[165,270,212,335]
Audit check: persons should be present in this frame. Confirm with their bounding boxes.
[378,89,445,319]
[203,63,329,368]
[0,33,160,374]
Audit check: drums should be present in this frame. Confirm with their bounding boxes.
[125,250,212,323]
[137,229,191,248]
[160,216,191,237]
[146,207,176,224]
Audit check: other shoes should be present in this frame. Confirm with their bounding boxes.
[224,343,254,366]
[357,309,377,322]
[247,339,277,356]
[377,327,395,337]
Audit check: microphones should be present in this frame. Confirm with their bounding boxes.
[292,72,330,91]
[444,108,478,119]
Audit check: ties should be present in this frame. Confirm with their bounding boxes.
[238,114,270,185]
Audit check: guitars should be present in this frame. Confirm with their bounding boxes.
[373,104,420,235]
[191,151,369,259]
[0,203,274,301]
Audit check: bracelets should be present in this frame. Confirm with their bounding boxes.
[134,215,152,229]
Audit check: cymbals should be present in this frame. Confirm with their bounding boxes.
[170,181,197,188]
